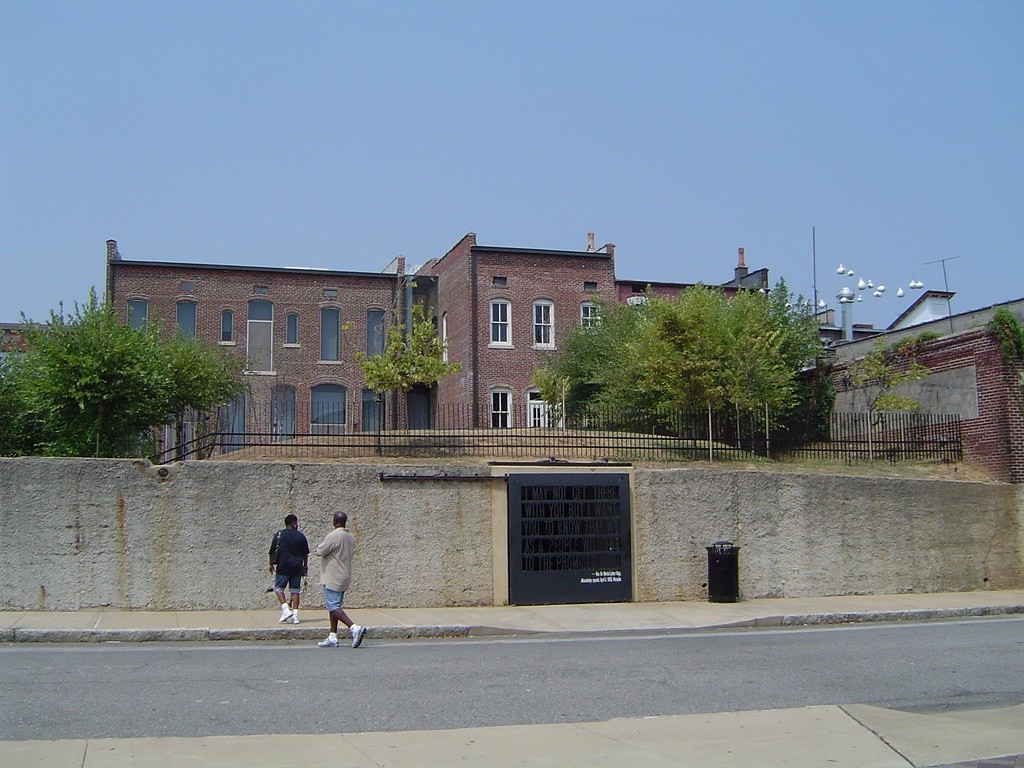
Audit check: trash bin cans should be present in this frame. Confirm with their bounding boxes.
[705,541,741,602]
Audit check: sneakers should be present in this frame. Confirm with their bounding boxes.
[352,626,366,648]
[318,637,338,647]
[282,615,299,624]
[278,610,293,622]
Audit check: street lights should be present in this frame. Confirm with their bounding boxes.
[783,299,827,344]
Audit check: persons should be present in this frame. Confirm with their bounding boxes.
[268,515,310,624]
[317,511,366,648]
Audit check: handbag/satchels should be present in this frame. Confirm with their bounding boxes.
[272,530,281,564]
